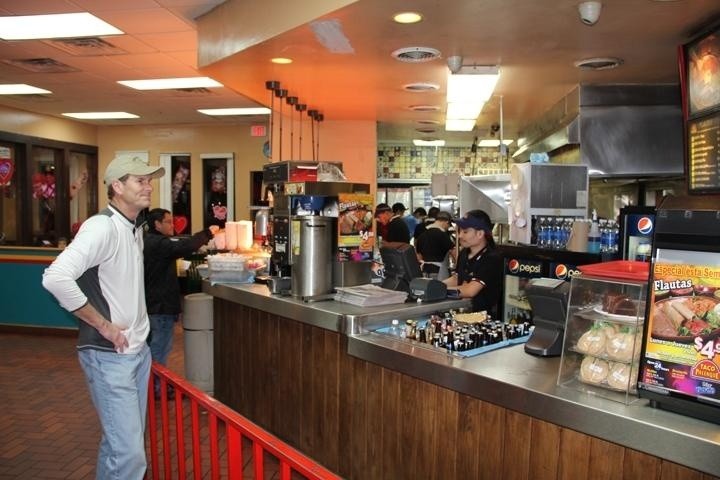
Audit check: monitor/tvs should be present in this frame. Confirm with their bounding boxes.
[378,242,423,295]
[524,278,571,356]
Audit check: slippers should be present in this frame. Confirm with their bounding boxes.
[151,389,185,400]
[167,384,175,388]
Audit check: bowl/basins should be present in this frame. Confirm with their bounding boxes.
[694,286,718,293]
[654,288,669,297]
[671,288,692,296]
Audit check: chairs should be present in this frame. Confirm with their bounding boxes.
[0,254,82,332]
[182,253,208,287]
[206,249,230,256]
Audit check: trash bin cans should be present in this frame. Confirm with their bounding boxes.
[182,293,213,394]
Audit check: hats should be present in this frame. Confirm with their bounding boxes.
[451,210,492,230]
[374,203,391,218]
[436,211,451,224]
[392,203,409,213]
[103,154,165,187]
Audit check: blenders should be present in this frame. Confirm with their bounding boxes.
[262,161,370,302]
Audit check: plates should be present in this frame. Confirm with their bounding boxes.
[652,296,719,340]
[594,300,646,322]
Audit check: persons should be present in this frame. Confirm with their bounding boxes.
[416,211,455,262]
[413,207,440,247]
[442,209,505,321]
[392,202,409,218]
[143,208,220,401]
[374,203,410,244]
[42,154,166,480]
[402,207,427,242]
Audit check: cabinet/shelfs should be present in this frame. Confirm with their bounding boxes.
[554,260,652,405]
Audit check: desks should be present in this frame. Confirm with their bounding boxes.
[202,276,720,480]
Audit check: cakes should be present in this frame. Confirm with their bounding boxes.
[602,291,638,317]
[655,289,669,301]
[670,286,695,296]
[695,284,716,295]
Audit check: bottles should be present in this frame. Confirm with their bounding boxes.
[599,229,605,251]
[514,324,518,331]
[497,324,502,329]
[464,324,468,328]
[457,326,461,330]
[495,320,500,325]
[516,328,521,337]
[498,328,503,341]
[555,225,562,248]
[468,324,482,349]
[509,330,515,339]
[604,229,609,252]
[460,334,466,340]
[506,326,512,337]
[462,329,467,333]
[459,337,465,343]
[547,226,553,246]
[538,225,545,245]
[405,314,445,347]
[458,342,464,351]
[504,323,509,332]
[523,322,529,335]
[388,320,400,337]
[455,330,460,339]
[608,228,614,252]
[447,330,455,350]
[445,317,453,330]
[482,322,497,342]
[614,229,619,252]
